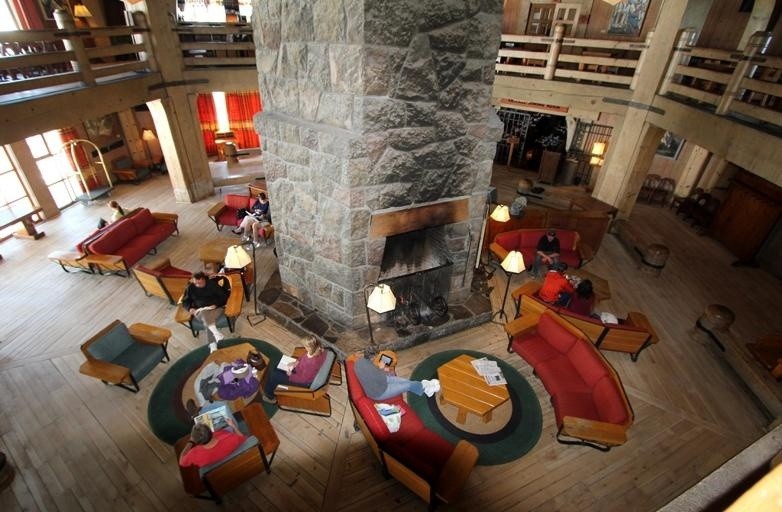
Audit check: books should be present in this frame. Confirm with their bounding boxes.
[193,406,230,435]
[194,305,216,316]
[471,357,507,386]
[277,355,297,372]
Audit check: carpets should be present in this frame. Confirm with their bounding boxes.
[148,337,291,446]
[408,350,543,465]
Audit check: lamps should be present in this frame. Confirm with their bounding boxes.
[142,127,159,172]
[363,283,396,345]
[74,2,92,17]
[491,247,526,325]
[224,241,266,327]
[482,202,511,279]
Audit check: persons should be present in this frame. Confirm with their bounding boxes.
[231,193,271,251]
[179,399,247,468]
[109,200,129,222]
[182,271,224,354]
[567,280,595,315]
[263,334,326,404]
[531,231,560,279]
[539,263,574,307]
[353,343,440,401]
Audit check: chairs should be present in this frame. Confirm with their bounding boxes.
[637,174,720,230]
[79,320,172,393]
[173,403,280,505]
[208,184,273,245]
[111,156,152,186]
[132,257,254,337]
[273,347,343,417]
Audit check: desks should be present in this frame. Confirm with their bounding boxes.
[0,205,45,260]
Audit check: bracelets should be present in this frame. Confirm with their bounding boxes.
[187,441,195,445]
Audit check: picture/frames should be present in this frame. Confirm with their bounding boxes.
[655,130,687,161]
[38,0,73,21]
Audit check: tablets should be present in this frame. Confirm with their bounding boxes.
[379,354,392,366]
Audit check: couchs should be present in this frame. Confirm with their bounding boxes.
[84,208,180,278]
[48,207,141,275]
[512,281,660,362]
[504,309,635,452]
[345,356,480,511]
[488,229,594,269]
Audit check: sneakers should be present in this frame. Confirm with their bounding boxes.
[231,229,260,251]
[208,333,224,354]
[262,394,277,404]
[420,379,440,398]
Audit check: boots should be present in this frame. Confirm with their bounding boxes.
[187,399,202,425]
